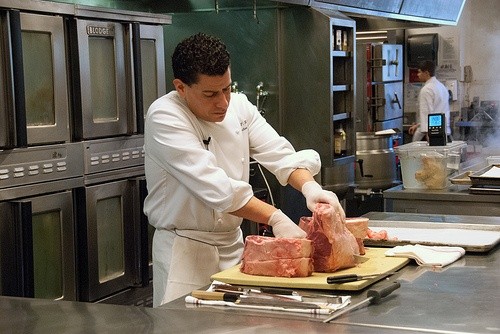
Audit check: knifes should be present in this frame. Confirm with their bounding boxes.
[326,271,401,284]
[190,290,321,310]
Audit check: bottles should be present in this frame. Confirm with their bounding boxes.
[335,122,347,158]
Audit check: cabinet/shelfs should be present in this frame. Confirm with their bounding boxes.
[275,8,357,169]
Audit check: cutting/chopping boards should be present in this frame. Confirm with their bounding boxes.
[211,244,411,291]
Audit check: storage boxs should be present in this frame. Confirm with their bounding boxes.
[394,140,467,191]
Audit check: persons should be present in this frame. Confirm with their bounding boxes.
[409,60,452,143]
[143,32,345,308]
